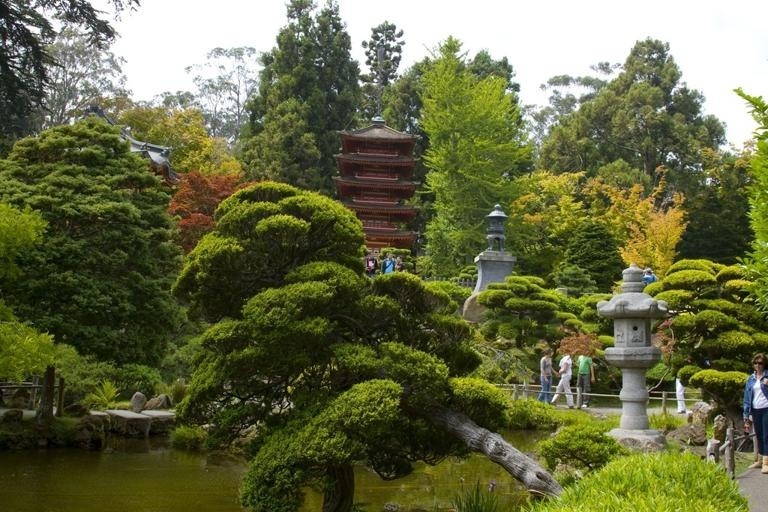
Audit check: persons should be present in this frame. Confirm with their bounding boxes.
[536,349,560,406]
[366,246,407,277]
[549,351,575,410]
[572,349,596,410]
[742,353,768,475]
[641,267,657,286]
[673,377,687,414]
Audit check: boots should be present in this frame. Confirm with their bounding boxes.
[749,454,768,474]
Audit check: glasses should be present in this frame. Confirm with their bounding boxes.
[754,361,763,365]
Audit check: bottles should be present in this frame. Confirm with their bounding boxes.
[743,419,751,437]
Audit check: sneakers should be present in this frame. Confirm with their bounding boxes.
[570,405,588,409]
